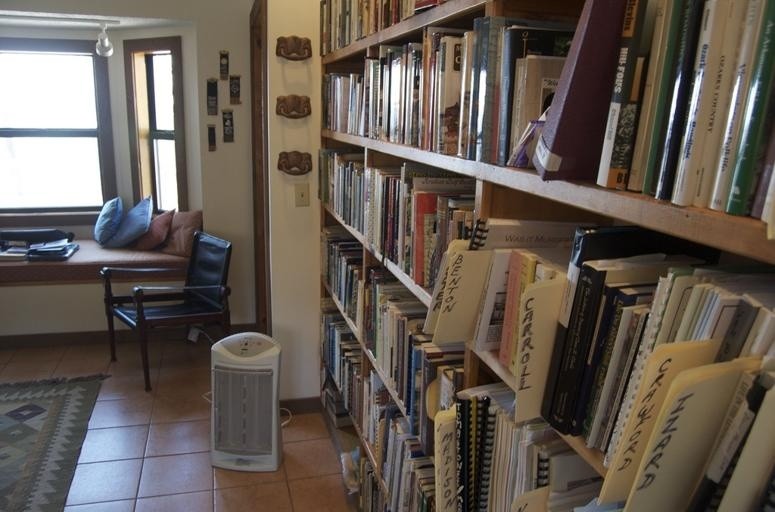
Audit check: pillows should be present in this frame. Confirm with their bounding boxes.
[92,196,123,245]
[107,195,153,248]
[129,209,177,251]
[164,210,203,257]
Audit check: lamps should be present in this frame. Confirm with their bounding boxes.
[94,22,115,59]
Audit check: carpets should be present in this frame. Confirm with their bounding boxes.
[0,373,110,512]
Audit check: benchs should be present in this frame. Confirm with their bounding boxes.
[0,206,203,352]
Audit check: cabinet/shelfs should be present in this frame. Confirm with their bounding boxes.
[318,1,774,511]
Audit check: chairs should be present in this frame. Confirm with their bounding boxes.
[99,230,234,392]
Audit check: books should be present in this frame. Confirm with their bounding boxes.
[318,3,775,512]
[0,239,80,261]
